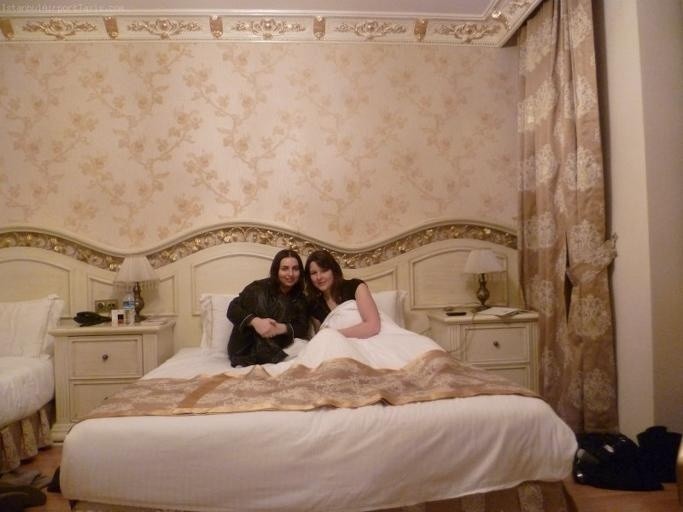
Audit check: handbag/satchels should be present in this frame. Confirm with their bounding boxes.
[572,430,666,493]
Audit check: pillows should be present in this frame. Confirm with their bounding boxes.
[196,289,409,356]
[0,293,65,357]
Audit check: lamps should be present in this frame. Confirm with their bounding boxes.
[460,246,508,313]
[111,253,160,322]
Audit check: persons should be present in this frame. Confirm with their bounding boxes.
[226,249,309,368]
[301,249,380,340]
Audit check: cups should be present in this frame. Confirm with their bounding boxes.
[110,309,124,323]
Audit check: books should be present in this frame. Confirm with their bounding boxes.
[477,305,529,318]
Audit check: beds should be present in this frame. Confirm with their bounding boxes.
[0,225,69,478]
[56,220,583,512]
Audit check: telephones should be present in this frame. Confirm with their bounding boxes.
[73,311,105,325]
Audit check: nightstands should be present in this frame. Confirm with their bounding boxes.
[425,310,541,395]
[45,317,179,444]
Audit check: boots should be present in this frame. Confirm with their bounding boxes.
[635,424,683,484]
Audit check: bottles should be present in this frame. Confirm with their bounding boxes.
[121,289,134,326]
